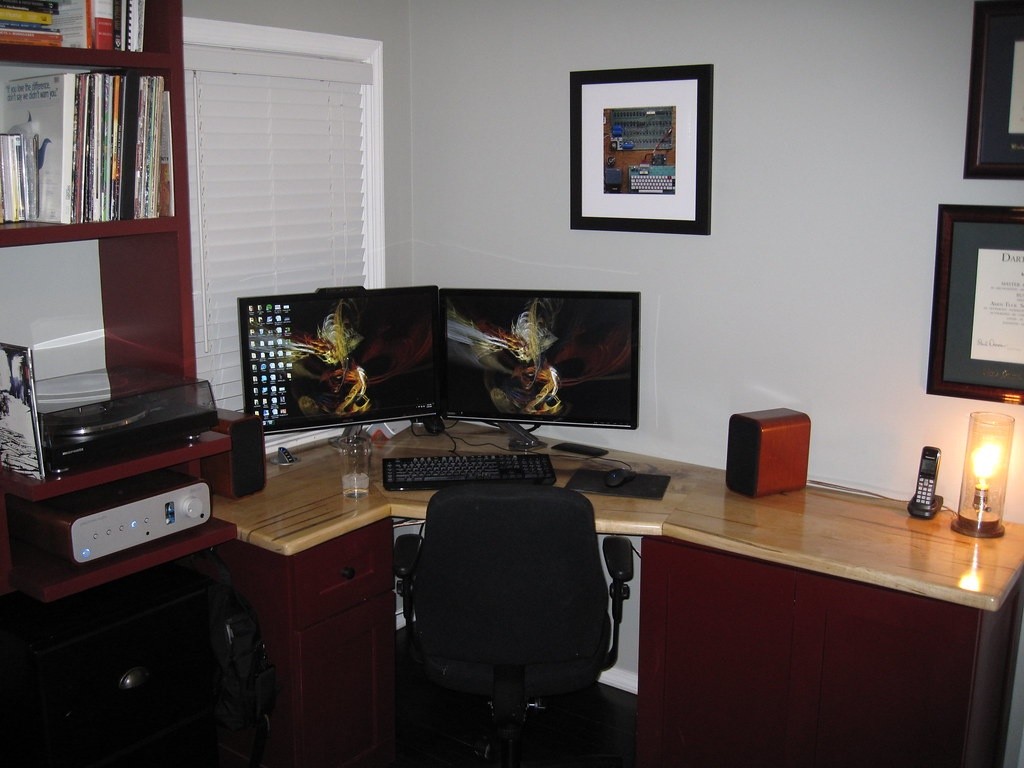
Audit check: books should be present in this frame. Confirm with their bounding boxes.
[0,71,175,226]
[0,0,146,53]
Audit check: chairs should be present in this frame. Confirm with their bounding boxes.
[392,482,634,768]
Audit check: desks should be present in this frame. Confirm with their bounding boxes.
[209,419,1024,768]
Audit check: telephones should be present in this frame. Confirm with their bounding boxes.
[907,445,944,518]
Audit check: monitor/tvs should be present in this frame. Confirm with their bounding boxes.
[236,285,439,451]
[438,288,642,451]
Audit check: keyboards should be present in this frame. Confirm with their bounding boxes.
[381,453,557,491]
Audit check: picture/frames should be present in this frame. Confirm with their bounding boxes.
[570,64,715,236]
[963,0,1024,180]
[925,204,1024,405]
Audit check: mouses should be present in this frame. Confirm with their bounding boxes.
[605,468,636,488]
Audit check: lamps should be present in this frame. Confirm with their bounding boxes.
[951,411,1015,538]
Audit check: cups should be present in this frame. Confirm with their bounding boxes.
[338,436,372,497]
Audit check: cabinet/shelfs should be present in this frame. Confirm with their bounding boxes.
[0,0,233,499]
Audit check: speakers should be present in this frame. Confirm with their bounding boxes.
[199,408,267,500]
[725,408,811,498]
